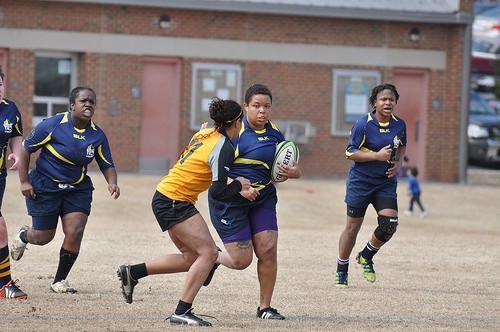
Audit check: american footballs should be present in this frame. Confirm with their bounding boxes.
[271,139,300,183]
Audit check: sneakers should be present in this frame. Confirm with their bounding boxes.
[335,272,348,288]
[203,246,222,286]
[170,308,212,326]
[51,282,77,293]
[257,307,285,320]
[11,225,29,261]
[0,280,27,299]
[116,264,138,304]
[356,252,377,283]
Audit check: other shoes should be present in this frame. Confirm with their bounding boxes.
[420,211,430,218]
[404,210,412,216]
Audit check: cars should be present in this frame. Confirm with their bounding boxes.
[467,91,500,168]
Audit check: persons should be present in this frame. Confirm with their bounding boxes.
[203,84,302,320]
[118,100,251,327]
[404,166,427,217]
[10,87,120,294]
[0,65,28,300]
[334,84,406,289]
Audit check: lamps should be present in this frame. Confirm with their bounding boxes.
[408,27,421,43]
[158,15,171,30]
